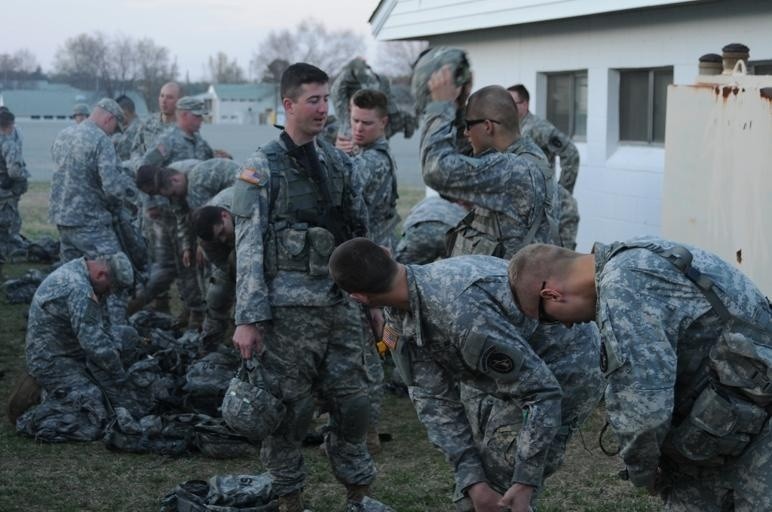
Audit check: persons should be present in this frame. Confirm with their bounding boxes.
[325,234,608,512]
[0,46,582,512]
[506,237,772,512]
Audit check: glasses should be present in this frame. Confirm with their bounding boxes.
[463,117,498,131]
[538,282,558,324]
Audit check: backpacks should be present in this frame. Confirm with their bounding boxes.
[329,58,415,140]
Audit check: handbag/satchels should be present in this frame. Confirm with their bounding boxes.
[89,358,172,417]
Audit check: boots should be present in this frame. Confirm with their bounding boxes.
[343,486,368,512]
[318,426,381,454]
[7,373,40,422]
[280,492,303,512]
[153,297,168,310]
[126,291,144,314]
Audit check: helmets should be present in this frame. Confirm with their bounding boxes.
[411,48,465,110]
[221,378,285,439]
[68,104,88,118]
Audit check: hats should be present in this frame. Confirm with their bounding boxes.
[176,97,207,115]
[105,252,134,298]
[97,97,126,134]
